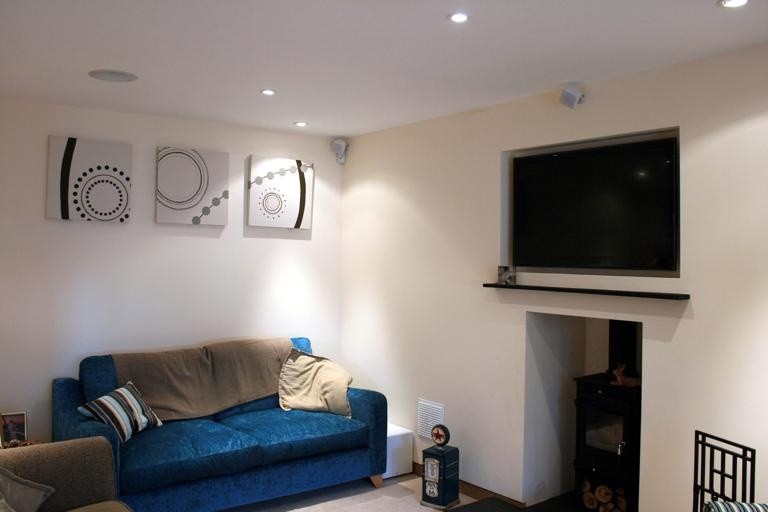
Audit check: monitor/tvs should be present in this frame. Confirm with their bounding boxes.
[512,136,677,271]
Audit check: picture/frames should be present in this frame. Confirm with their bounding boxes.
[0,411,28,446]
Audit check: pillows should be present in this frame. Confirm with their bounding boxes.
[278,347,353,420]
[76,379,163,446]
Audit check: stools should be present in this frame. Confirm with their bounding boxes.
[378,421,414,479]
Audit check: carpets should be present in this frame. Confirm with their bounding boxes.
[445,497,592,511]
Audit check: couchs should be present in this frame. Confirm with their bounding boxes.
[51,336,387,511]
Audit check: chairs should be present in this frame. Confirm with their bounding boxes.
[0,436,133,512]
